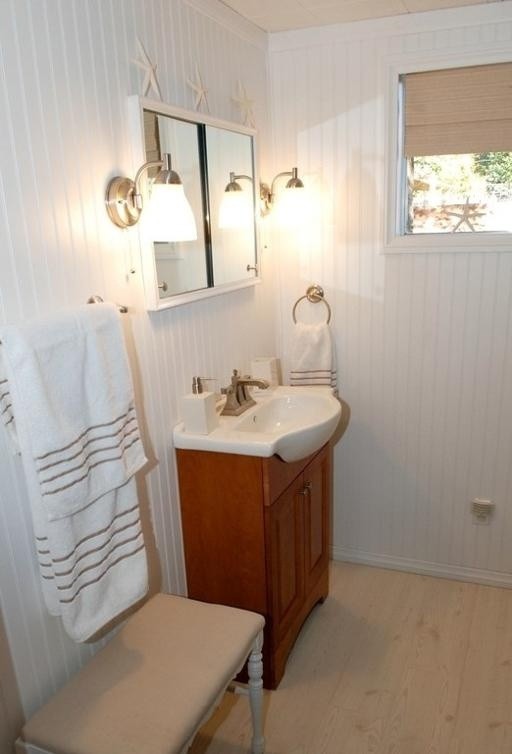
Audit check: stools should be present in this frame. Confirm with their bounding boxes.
[15,592,267,754]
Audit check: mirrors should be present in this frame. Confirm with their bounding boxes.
[126,94,262,312]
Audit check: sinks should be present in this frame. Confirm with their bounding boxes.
[220,391,342,458]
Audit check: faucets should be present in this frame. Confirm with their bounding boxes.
[220,368,271,415]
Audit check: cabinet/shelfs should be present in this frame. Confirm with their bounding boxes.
[264,441,333,653]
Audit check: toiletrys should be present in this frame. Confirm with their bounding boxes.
[183,377,216,436]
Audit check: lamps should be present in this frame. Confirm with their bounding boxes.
[259,165,319,232]
[218,170,253,230]
[105,149,198,244]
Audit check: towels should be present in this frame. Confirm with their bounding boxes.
[287,319,339,394]
[0,300,151,642]
